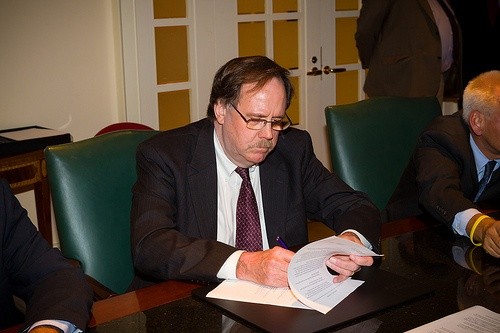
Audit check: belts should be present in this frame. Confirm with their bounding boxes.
[443,64,457,78]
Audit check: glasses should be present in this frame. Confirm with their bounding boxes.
[228,100,292,131]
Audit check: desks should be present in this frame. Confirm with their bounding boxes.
[0,149,53,248]
[0,214,500,333]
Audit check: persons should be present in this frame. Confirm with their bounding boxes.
[131,56,384,288]
[354,0,462,115]
[384,70,500,257]
[0,174,90,332]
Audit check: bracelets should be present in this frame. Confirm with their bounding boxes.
[469,215,491,246]
[470,247,482,275]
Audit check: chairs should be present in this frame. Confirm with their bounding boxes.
[43,130,163,294]
[325,95,442,242]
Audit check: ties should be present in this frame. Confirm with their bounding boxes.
[472,160,496,204]
[235,166,263,251]
[437,0,461,65]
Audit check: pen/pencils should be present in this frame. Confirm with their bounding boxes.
[276,236,287,249]
